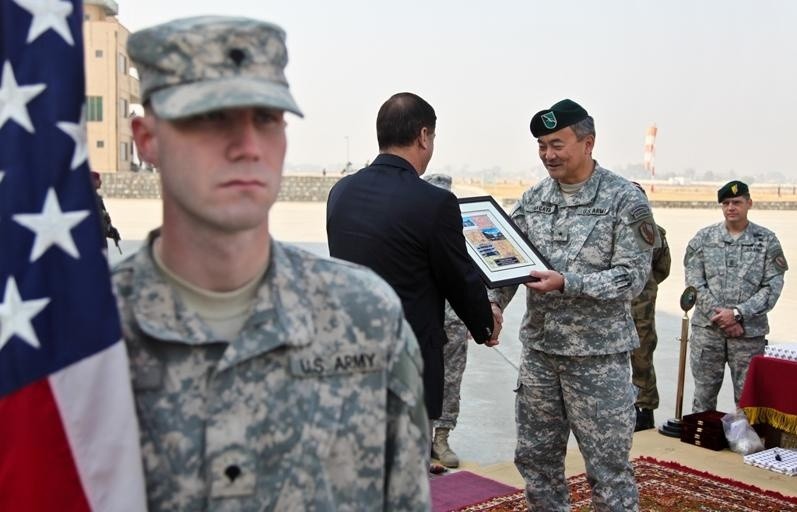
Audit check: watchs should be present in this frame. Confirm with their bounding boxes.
[733,307,744,324]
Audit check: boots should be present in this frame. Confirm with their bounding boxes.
[633,406,655,432]
[432,429,460,468]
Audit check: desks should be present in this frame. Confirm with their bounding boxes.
[735,354,797,450]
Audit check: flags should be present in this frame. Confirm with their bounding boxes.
[0,0,150,512]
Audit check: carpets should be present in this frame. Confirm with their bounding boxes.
[429,470,520,512]
[443,455,797,512]
[446,425,797,497]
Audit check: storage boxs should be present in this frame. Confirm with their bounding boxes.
[680,408,742,452]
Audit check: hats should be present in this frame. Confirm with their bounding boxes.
[126,12,305,120]
[530,98,590,139]
[717,180,750,204]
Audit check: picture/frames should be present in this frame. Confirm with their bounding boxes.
[457,195,555,291]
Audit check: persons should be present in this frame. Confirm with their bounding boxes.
[631,180,672,432]
[421,173,473,468]
[110,13,433,512]
[91,172,112,231]
[325,92,503,437]
[684,180,789,414]
[484,97,663,512]
